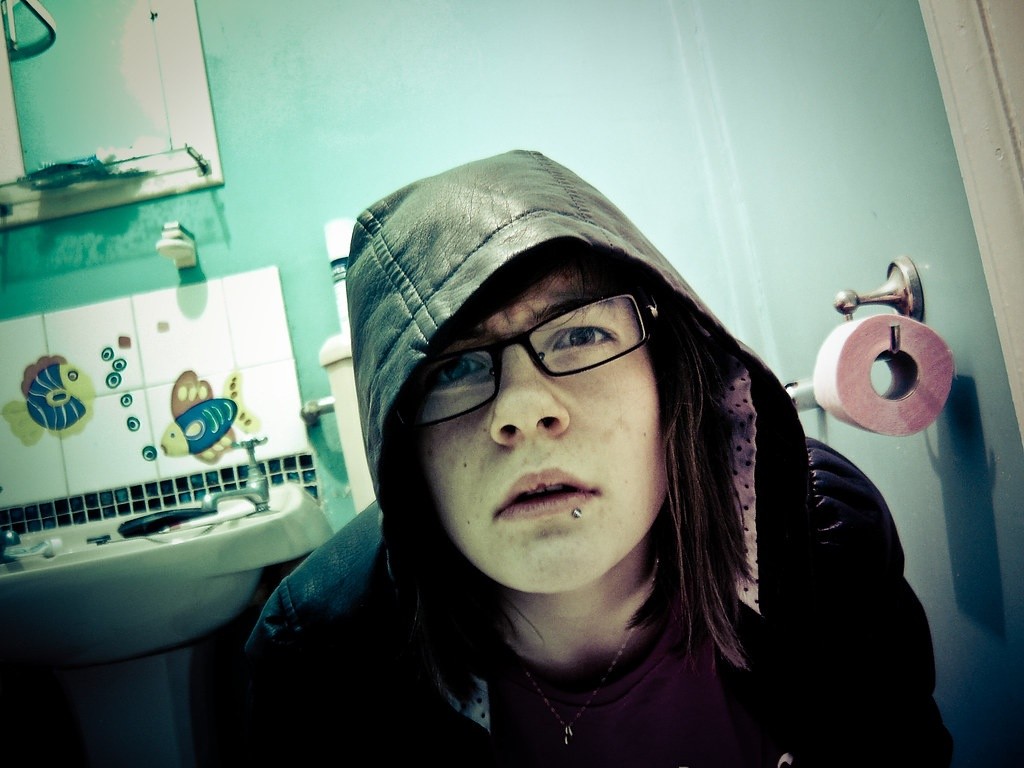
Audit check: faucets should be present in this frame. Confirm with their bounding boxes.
[197,430,278,519]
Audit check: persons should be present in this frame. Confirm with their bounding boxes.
[243,149,953,768]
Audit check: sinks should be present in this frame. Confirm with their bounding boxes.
[2,479,337,661]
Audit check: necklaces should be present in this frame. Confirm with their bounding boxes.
[521,556,661,748]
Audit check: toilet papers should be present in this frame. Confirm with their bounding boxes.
[811,311,956,438]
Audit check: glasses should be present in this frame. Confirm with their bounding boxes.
[392,289,659,434]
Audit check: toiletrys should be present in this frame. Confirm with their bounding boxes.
[3,538,63,559]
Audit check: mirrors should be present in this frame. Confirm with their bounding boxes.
[1,0,173,177]
